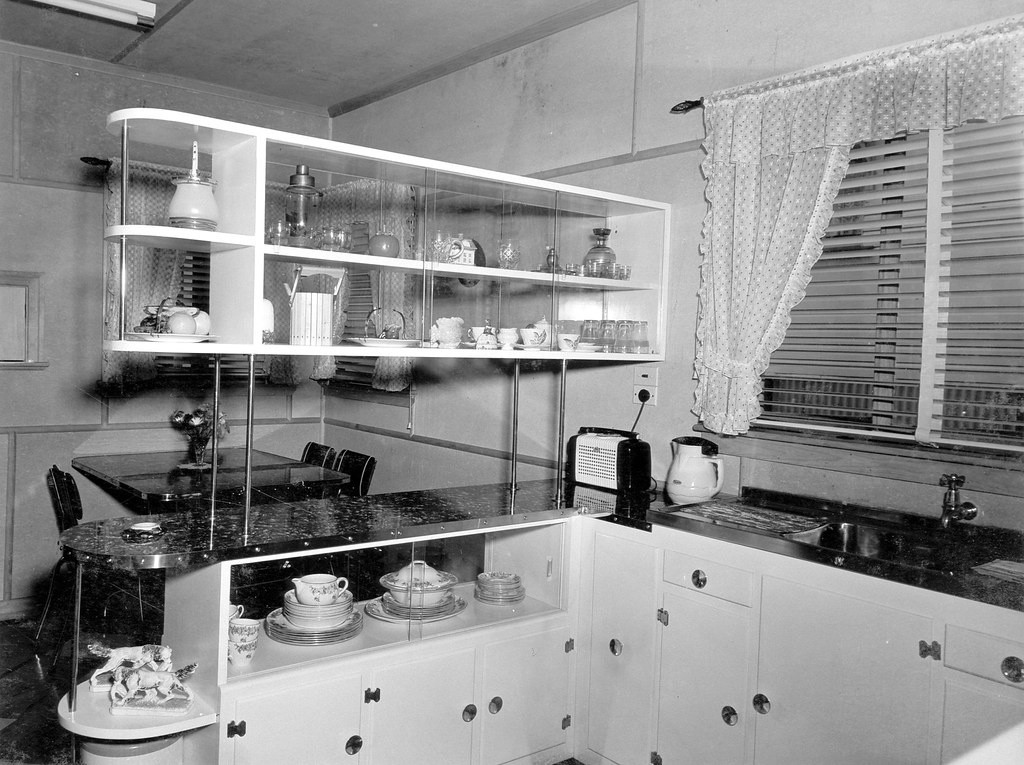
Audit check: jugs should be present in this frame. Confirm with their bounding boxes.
[665,436,724,505]
[292,574,349,606]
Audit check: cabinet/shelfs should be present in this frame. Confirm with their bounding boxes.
[103,108,672,516]
[165,516,1024,764]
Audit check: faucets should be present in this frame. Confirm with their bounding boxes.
[939,473,979,531]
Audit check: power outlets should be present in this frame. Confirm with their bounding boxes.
[631,385,658,404]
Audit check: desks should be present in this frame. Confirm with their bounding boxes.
[72,446,351,515]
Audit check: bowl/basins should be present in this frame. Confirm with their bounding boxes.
[283,588,354,629]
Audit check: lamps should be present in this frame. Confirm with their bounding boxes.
[18,0,160,31]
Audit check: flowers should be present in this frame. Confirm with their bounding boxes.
[171,402,231,440]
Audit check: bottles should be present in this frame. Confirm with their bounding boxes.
[583,228,616,263]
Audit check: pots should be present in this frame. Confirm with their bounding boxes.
[379,560,458,607]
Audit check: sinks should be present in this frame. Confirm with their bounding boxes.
[779,517,991,582]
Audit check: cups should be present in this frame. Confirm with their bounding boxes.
[496,239,522,270]
[497,328,519,349]
[566,259,632,280]
[265,219,353,252]
[467,326,501,343]
[426,229,464,262]
[228,605,261,668]
[557,333,581,352]
[520,329,547,346]
[579,320,650,354]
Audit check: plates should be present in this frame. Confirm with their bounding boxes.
[474,571,526,607]
[580,345,603,352]
[577,342,596,347]
[365,591,468,625]
[346,337,421,348]
[463,342,476,348]
[264,607,364,646]
[124,331,220,343]
[515,343,547,350]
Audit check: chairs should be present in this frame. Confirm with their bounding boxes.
[300,441,337,470]
[331,449,377,498]
[36,464,110,672]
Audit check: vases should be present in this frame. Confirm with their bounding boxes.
[190,437,208,456]
